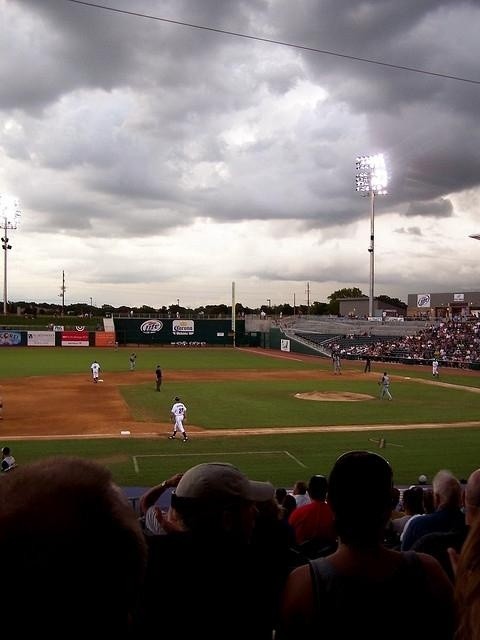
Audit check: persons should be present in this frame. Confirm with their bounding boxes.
[0,447,480,640]
[378,371,392,401]
[129,352,136,370]
[128,311,130,317]
[198,310,204,319]
[0,333,19,344]
[115,340,118,352]
[237,311,241,318]
[168,396,188,443]
[297,307,480,376]
[176,310,180,318]
[261,309,264,320]
[48,311,111,332]
[280,310,283,319]
[178,337,209,348]
[241,310,244,317]
[168,309,171,318]
[155,365,163,392]
[91,360,100,383]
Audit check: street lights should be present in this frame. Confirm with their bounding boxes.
[0,194,21,316]
[355,152,389,318]
[468,233,480,240]
[267,298,271,308]
[176,299,180,308]
[89,296,93,306]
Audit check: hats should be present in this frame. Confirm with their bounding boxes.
[171,463,275,514]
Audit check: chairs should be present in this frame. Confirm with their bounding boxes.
[407,531,467,578]
[312,575,458,640]
[295,333,479,364]
